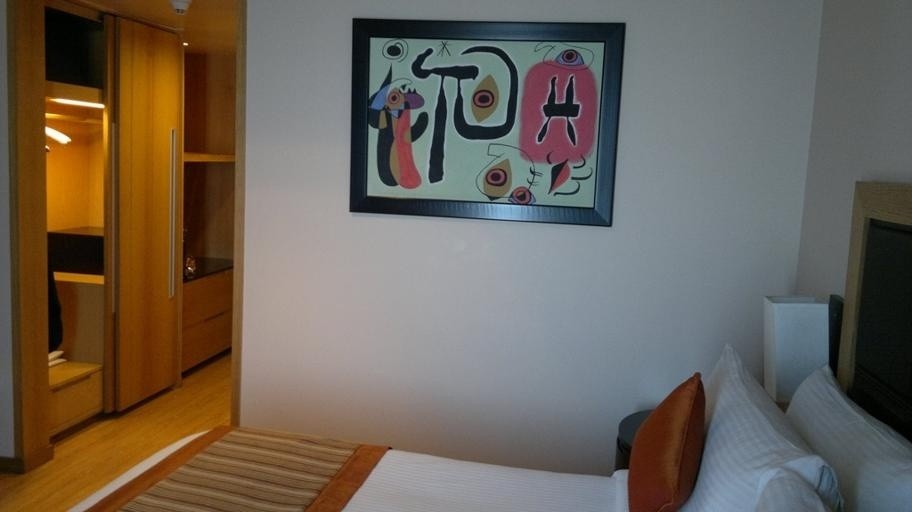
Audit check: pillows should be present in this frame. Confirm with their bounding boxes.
[627,345,912,511]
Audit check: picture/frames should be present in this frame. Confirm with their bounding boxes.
[349,18,626,228]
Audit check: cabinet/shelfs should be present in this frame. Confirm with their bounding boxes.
[7,0,235,473]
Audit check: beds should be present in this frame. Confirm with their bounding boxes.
[69,427,626,512]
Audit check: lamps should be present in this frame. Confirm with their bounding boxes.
[763,296,836,412]
